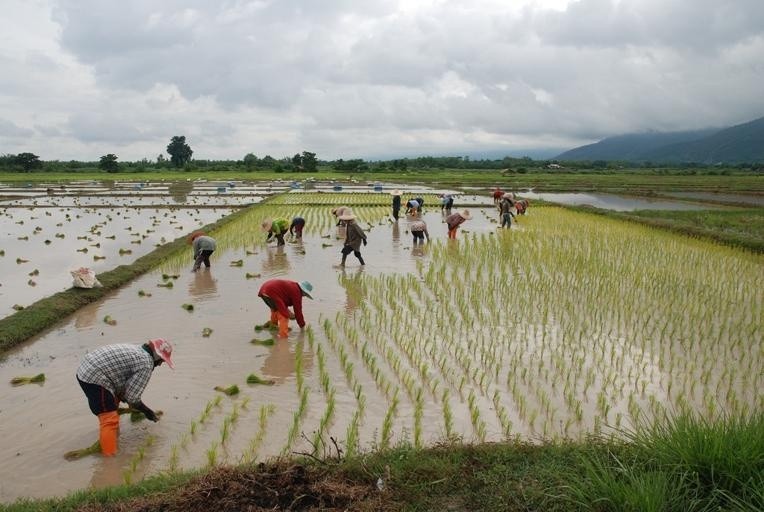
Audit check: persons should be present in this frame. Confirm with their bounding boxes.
[392,189,400,220]
[438,192,453,209]
[410,220,429,246]
[331,206,349,227]
[260,217,290,246]
[446,209,473,239]
[491,191,529,230]
[191,231,217,273]
[290,216,305,237]
[76,337,176,456]
[407,198,423,216]
[258,279,313,337]
[338,209,368,266]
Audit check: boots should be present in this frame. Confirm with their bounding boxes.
[272,308,288,338]
[97,411,120,455]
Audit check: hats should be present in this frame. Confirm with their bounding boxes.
[297,279,314,301]
[331,208,336,213]
[459,208,474,220]
[338,208,356,221]
[149,338,175,368]
[390,189,404,196]
[187,231,206,244]
[260,218,272,232]
